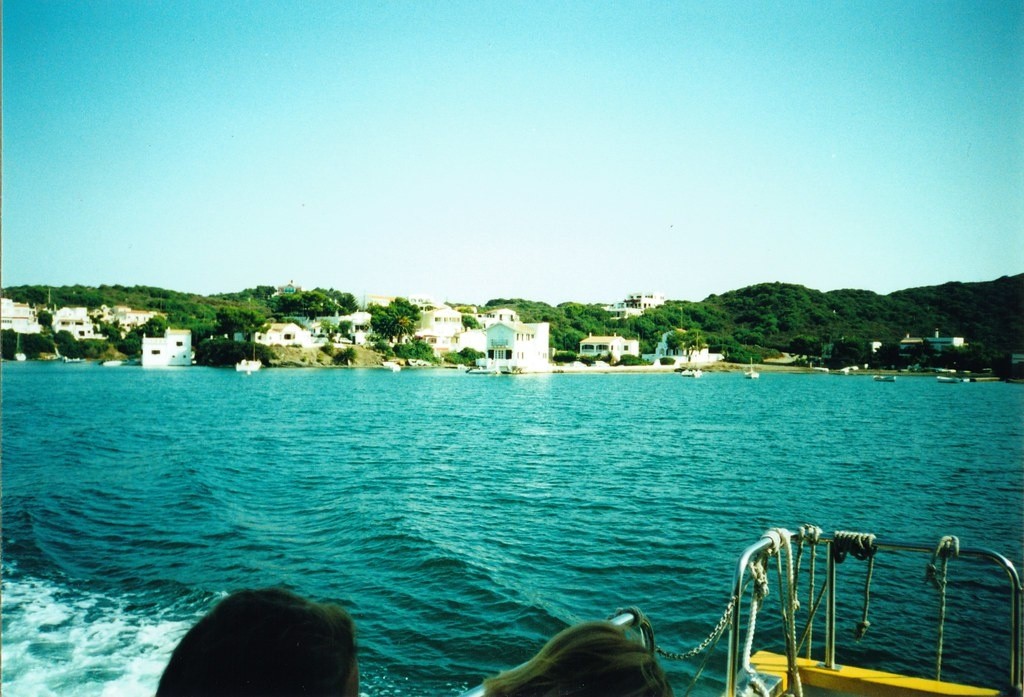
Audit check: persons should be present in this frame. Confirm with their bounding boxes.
[154,587,360,697]
[481,619,675,697]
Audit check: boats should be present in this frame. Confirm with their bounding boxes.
[384,361,403,372]
[679,355,969,383]
[235,360,263,372]
[466,365,525,376]
[13,352,141,366]
